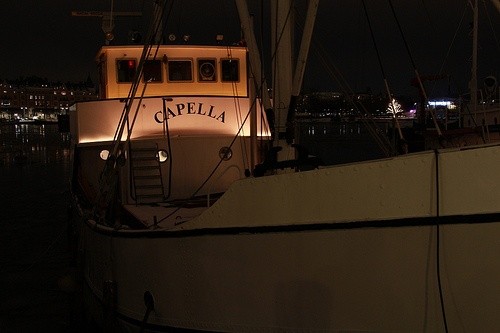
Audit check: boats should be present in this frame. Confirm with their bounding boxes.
[368,0,499,134]
[384,121,500,152]
[60,0,500,333]
